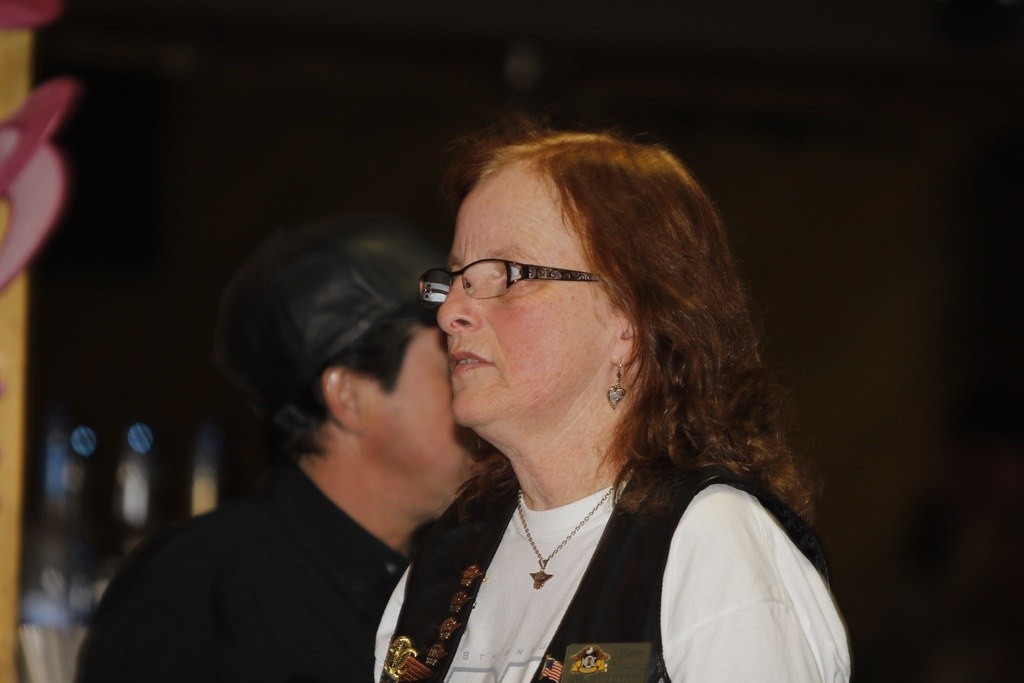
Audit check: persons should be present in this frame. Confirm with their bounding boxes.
[74,212,494,682]
[373,131,853,683]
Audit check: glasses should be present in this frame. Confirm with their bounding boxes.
[417,259,602,310]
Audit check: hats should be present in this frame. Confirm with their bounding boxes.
[215,217,450,406]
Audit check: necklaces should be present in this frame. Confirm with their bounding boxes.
[516,485,617,589]
